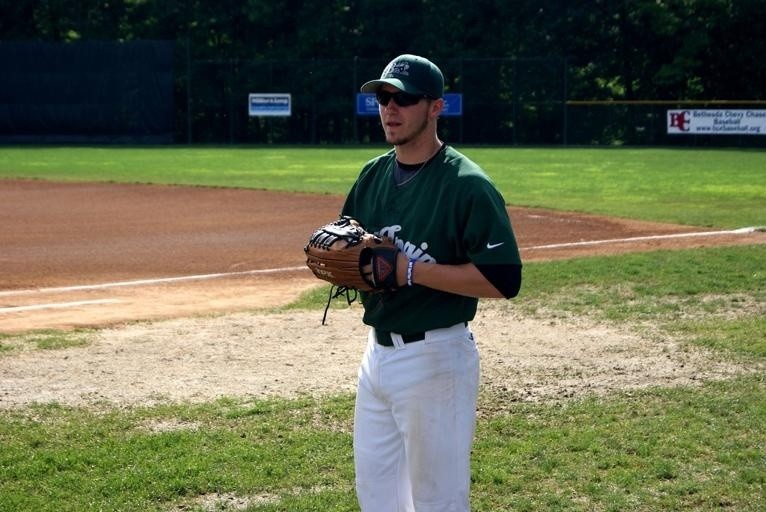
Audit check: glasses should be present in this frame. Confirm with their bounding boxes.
[377,90,424,106]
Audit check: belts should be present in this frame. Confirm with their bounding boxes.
[376,330,424,346]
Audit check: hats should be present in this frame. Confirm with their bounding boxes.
[361,54,444,99]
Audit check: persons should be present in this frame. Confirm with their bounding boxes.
[304,54,522,512]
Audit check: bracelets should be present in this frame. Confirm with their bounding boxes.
[407,258,416,286]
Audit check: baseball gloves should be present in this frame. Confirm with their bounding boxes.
[304,215,402,293]
